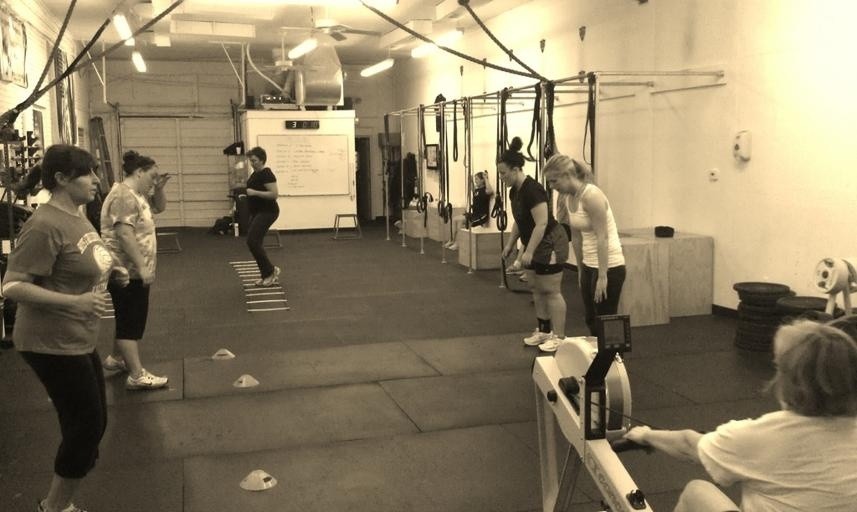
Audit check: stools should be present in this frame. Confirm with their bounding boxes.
[330,214,362,241]
[264,230,285,248]
[157,233,182,254]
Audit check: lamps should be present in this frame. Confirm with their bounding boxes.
[111,9,153,78]
[288,25,462,78]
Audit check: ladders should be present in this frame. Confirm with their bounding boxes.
[90,117,114,193]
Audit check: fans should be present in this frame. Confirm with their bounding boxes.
[279,3,382,43]
[240,36,332,77]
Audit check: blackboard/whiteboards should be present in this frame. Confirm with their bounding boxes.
[257,135,349,196]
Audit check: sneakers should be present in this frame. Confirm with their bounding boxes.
[524,328,568,352]
[505,264,528,282]
[101,355,128,371]
[124,368,168,390]
[255,266,281,287]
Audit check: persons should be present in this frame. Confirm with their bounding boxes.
[237,148,281,286]
[622,319,857,512]
[496,136,569,353]
[0,143,130,512]
[442,169,494,251]
[99,150,172,390]
[541,154,627,336]
